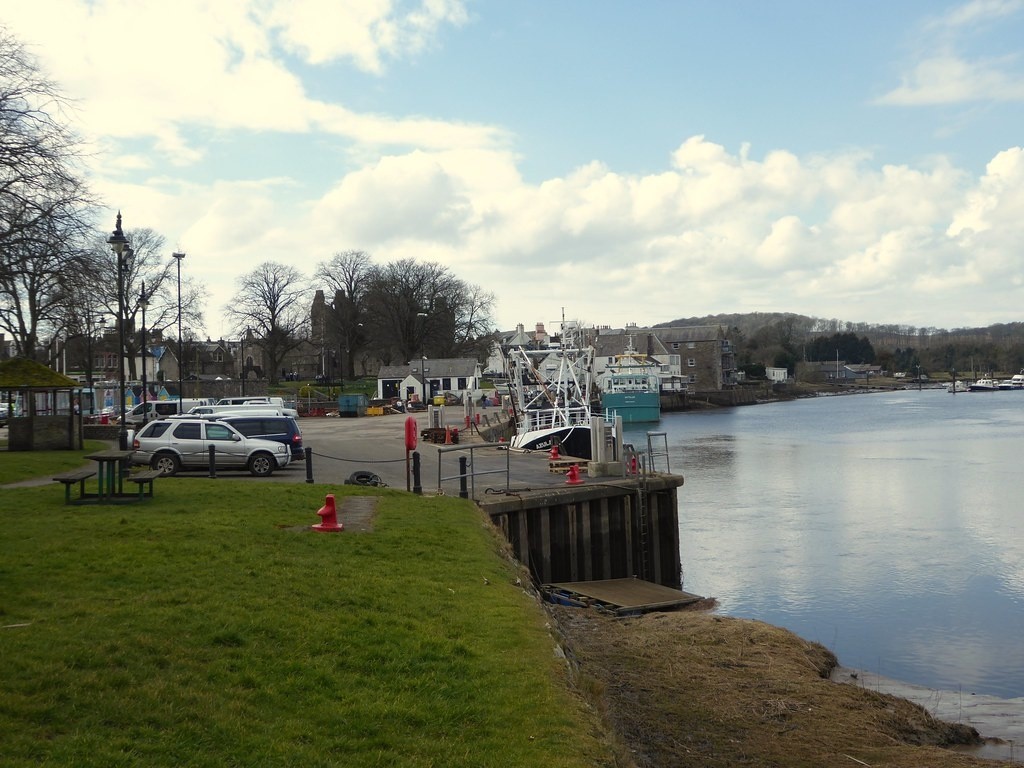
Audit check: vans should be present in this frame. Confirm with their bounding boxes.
[187,397,300,420]
[116,397,213,425]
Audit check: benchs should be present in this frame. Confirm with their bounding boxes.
[52,470,97,505]
[127,470,164,503]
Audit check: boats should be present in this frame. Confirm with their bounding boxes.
[912,375,929,383]
[998,375,1024,389]
[601,336,661,423]
[969,379,999,391]
[947,381,968,393]
[507,307,624,459]
[894,373,905,379]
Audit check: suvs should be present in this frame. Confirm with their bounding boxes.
[126,417,292,477]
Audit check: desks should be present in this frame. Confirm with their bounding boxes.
[70,450,149,505]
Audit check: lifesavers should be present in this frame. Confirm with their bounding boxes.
[404,415,418,451]
[350,471,378,486]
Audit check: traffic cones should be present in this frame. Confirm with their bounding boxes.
[444,424,455,445]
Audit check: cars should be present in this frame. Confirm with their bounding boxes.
[208,416,305,462]
[0,403,17,419]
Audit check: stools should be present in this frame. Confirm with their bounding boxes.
[645,430,672,476]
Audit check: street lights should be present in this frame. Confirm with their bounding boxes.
[106,210,134,478]
[173,253,185,413]
[138,280,148,426]
[417,313,428,404]
